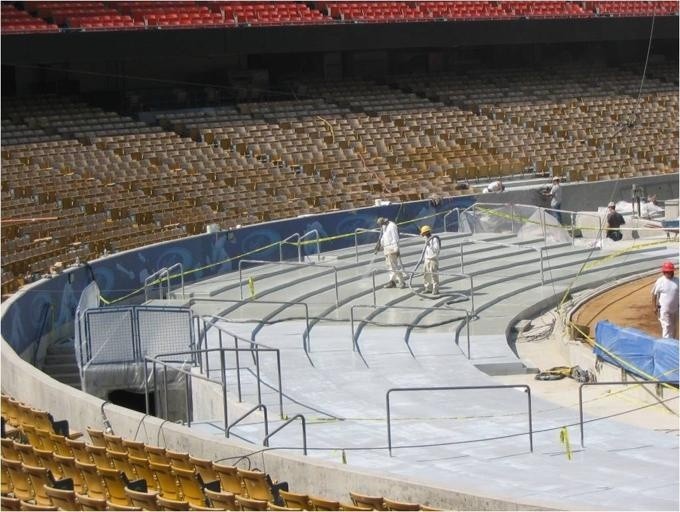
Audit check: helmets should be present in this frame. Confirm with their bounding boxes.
[552,175,560,181]
[608,201,615,207]
[420,225,431,234]
[661,261,675,272]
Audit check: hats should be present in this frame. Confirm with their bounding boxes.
[377,216,388,224]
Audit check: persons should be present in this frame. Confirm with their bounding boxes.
[607,202,625,241]
[420,225,440,295]
[377,217,405,289]
[482,181,505,194]
[651,261,679,338]
[542,176,562,224]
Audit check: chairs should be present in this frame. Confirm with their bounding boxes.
[1,392,446,510]
[0,5,680,320]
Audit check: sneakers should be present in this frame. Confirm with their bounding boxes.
[422,289,438,295]
[384,283,405,288]
